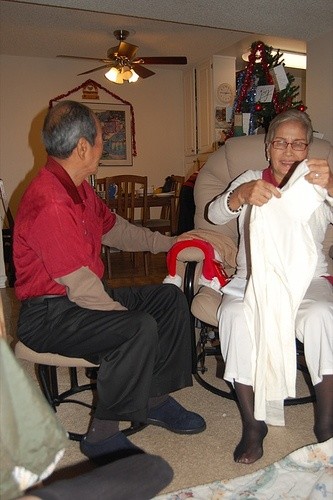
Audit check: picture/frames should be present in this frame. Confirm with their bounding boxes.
[51,101,132,166]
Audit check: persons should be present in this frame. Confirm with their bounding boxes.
[208,108,333,464]
[12,101,208,462]
[0,298,174,500]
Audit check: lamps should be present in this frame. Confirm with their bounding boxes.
[104,30,140,84]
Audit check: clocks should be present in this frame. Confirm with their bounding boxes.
[216,83,235,105]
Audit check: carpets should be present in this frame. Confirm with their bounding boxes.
[13,325,318,497]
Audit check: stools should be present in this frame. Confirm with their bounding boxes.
[13,341,151,440]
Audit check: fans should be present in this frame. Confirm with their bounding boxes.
[55,30,187,79]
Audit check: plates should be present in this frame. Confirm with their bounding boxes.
[138,193,175,197]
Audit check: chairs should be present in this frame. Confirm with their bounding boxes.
[92,135,333,405]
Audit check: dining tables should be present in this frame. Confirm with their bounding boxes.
[98,194,174,267]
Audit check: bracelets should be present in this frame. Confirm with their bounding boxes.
[227,190,243,212]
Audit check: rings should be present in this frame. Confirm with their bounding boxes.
[316,174,319,176]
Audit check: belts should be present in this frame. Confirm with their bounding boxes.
[21,295,63,305]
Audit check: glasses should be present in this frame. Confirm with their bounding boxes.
[271,140,309,151]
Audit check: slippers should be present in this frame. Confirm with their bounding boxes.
[80,430,147,466]
[142,395,207,434]
